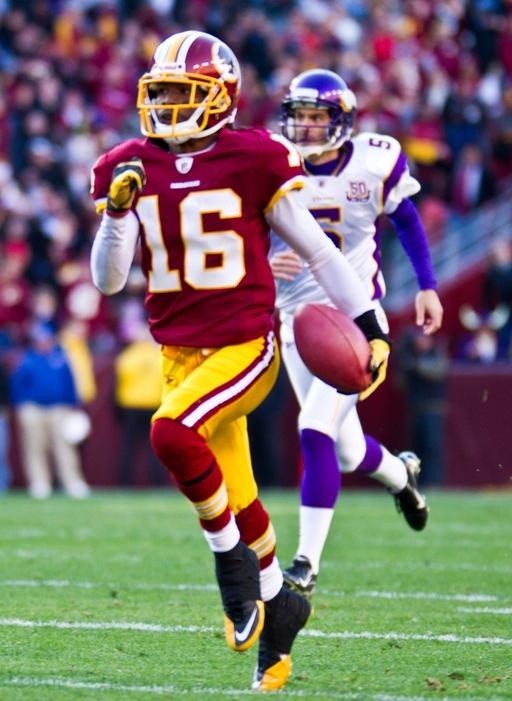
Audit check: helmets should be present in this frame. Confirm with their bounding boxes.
[136,30,242,145]
[278,68,357,160]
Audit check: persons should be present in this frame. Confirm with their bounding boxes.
[256,69,443,600]
[112,323,168,485]
[2,320,97,497]
[398,328,453,490]
[90,30,392,692]
[247,349,292,488]
[1,0,512,367]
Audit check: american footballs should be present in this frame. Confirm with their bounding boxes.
[294,305,371,390]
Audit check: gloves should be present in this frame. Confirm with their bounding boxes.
[106,156,148,211]
[337,310,394,401]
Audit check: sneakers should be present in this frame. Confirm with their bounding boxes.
[214,542,265,651]
[251,586,315,691]
[387,451,430,531]
[282,555,317,600]
